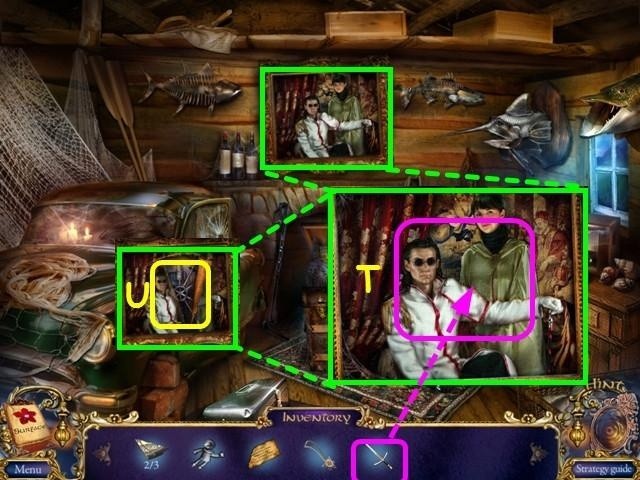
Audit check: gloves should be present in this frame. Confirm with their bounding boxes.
[540,296,564,315]
[364,118,373,128]
[212,295,222,303]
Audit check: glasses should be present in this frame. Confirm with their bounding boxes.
[410,258,437,266]
[305,104,319,108]
[156,279,167,283]
